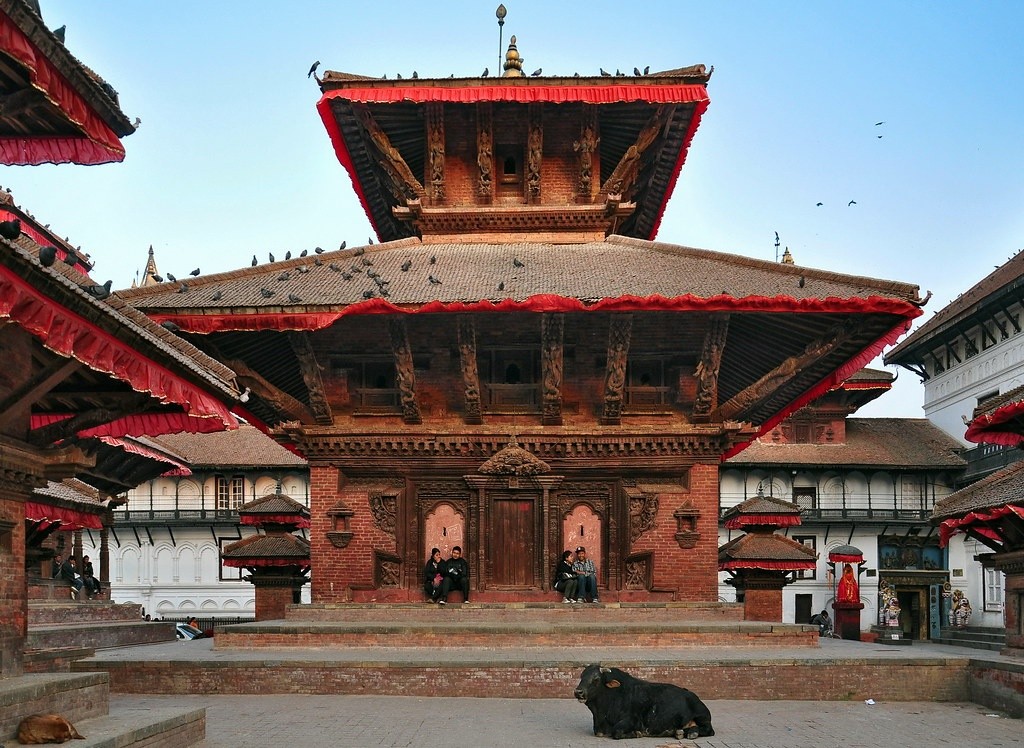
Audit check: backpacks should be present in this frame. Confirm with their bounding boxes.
[808,614,822,624]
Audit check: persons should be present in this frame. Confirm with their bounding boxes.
[190,617,199,639]
[812,610,832,637]
[144,614,151,622]
[424,546,470,604]
[52,554,101,601]
[553,546,599,604]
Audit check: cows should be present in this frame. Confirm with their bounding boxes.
[574,664,715,740]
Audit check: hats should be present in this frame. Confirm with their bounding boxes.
[575,546,585,553]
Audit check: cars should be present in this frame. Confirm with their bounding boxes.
[176,623,204,640]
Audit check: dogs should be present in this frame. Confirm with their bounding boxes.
[16,714,86,744]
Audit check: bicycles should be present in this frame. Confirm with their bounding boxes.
[823,623,841,639]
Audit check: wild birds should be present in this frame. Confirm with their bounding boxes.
[53,24,66,44]
[162,320,180,333]
[817,200,858,207]
[875,122,885,140]
[308,60,651,78]
[798,275,806,289]
[881,292,963,355]
[1,218,114,302]
[150,238,526,304]
[993,248,1021,268]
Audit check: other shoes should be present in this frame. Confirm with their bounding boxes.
[577,598,584,603]
[569,598,576,603]
[71,587,79,593]
[593,598,597,602]
[439,600,446,605]
[464,600,469,604]
[562,598,570,603]
[428,598,434,604]
[71,591,75,600]
[94,589,97,593]
